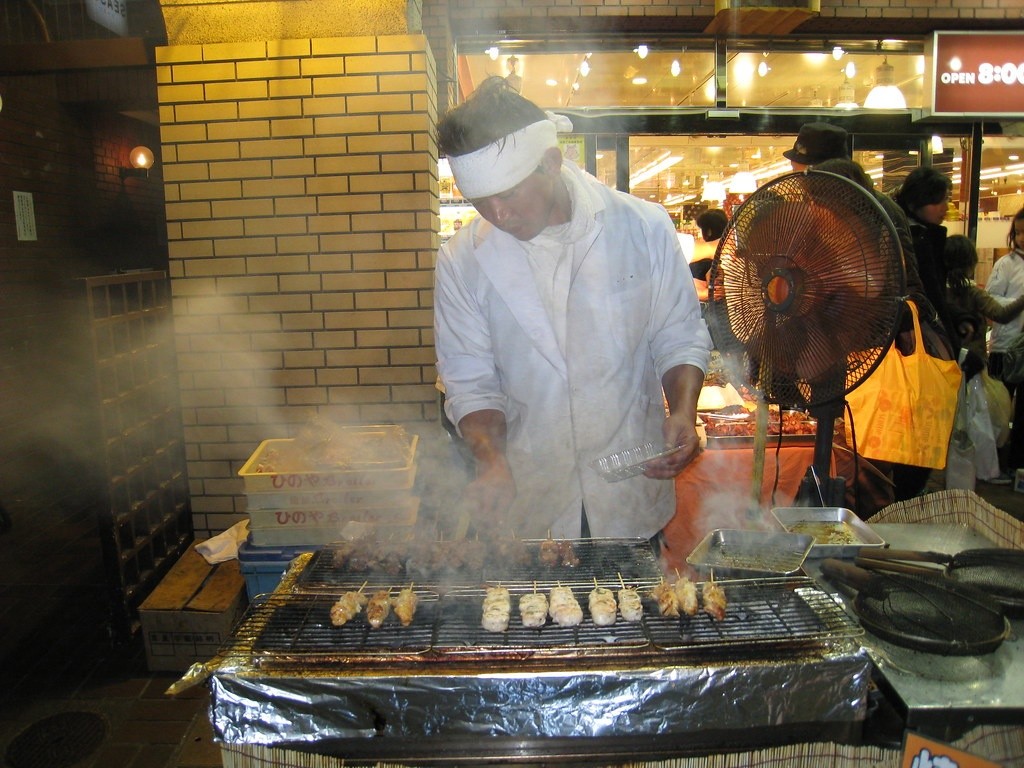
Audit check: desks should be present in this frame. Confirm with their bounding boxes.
[657,445,838,581]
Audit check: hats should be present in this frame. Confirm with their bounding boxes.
[783,122,850,165]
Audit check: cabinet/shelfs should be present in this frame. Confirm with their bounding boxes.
[74,269,191,655]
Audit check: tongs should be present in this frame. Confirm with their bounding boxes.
[697,411,753,422]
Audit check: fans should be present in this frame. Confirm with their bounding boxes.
[708,170,908,508]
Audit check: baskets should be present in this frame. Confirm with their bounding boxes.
[864,488,1024,550]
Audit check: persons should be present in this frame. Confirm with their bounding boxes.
[431,75,713,563]
[696,123,1024,504]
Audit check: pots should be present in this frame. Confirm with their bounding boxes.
[819,558,1011,655]
[860,548,1024,608]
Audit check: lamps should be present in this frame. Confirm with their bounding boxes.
[834,75,859,107]
[863,55,907,108]
[119,146,155,182]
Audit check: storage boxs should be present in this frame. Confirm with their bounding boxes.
[237,433,421,545]
[137,538,247,672]
[238,531,317,603]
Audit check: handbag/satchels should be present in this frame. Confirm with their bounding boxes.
[945,363,1014,491]
[844,300,963,470]
